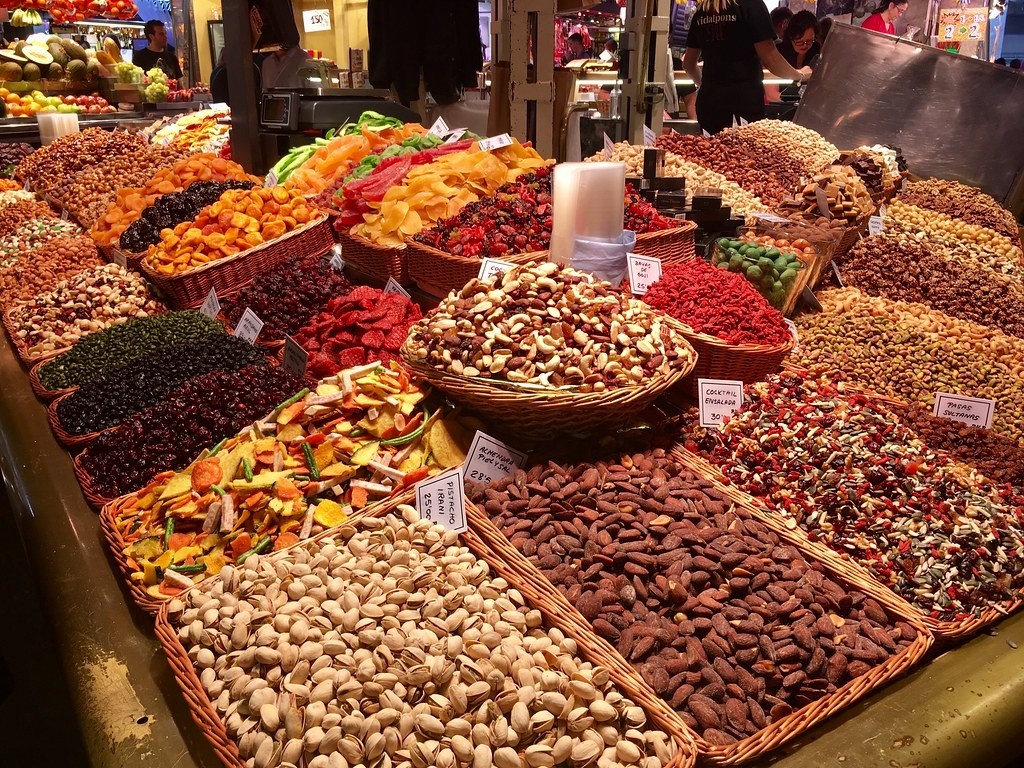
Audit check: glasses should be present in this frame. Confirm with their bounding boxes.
[895,5,905,14]
[791,37,816,47]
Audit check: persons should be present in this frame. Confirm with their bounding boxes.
[774,9,822,72]
[683,0,813,134]
[597,56,700,121]
[262,45,316,89]
[132,19,184,90]
[861,0,909,37]
[568,32,590,59]
[768,6,794,50]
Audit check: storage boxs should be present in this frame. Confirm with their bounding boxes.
[156,100,204,110]
[113,82,153,104]
[193,93,214,103]
[97,77,119,103]
[0,107,146,125]
[710,206,847,317]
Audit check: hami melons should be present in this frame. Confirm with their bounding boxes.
[0,32,96,80]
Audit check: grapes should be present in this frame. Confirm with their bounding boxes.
[116,61,169,103]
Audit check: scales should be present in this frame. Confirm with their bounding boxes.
[257,83,424,137]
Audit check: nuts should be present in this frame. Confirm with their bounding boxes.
[166,431,918,768]
[584,118,1024,441]
[1,127,204,357]
[400,262,690,393]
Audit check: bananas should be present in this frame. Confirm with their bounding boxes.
[9,9,43,27]
